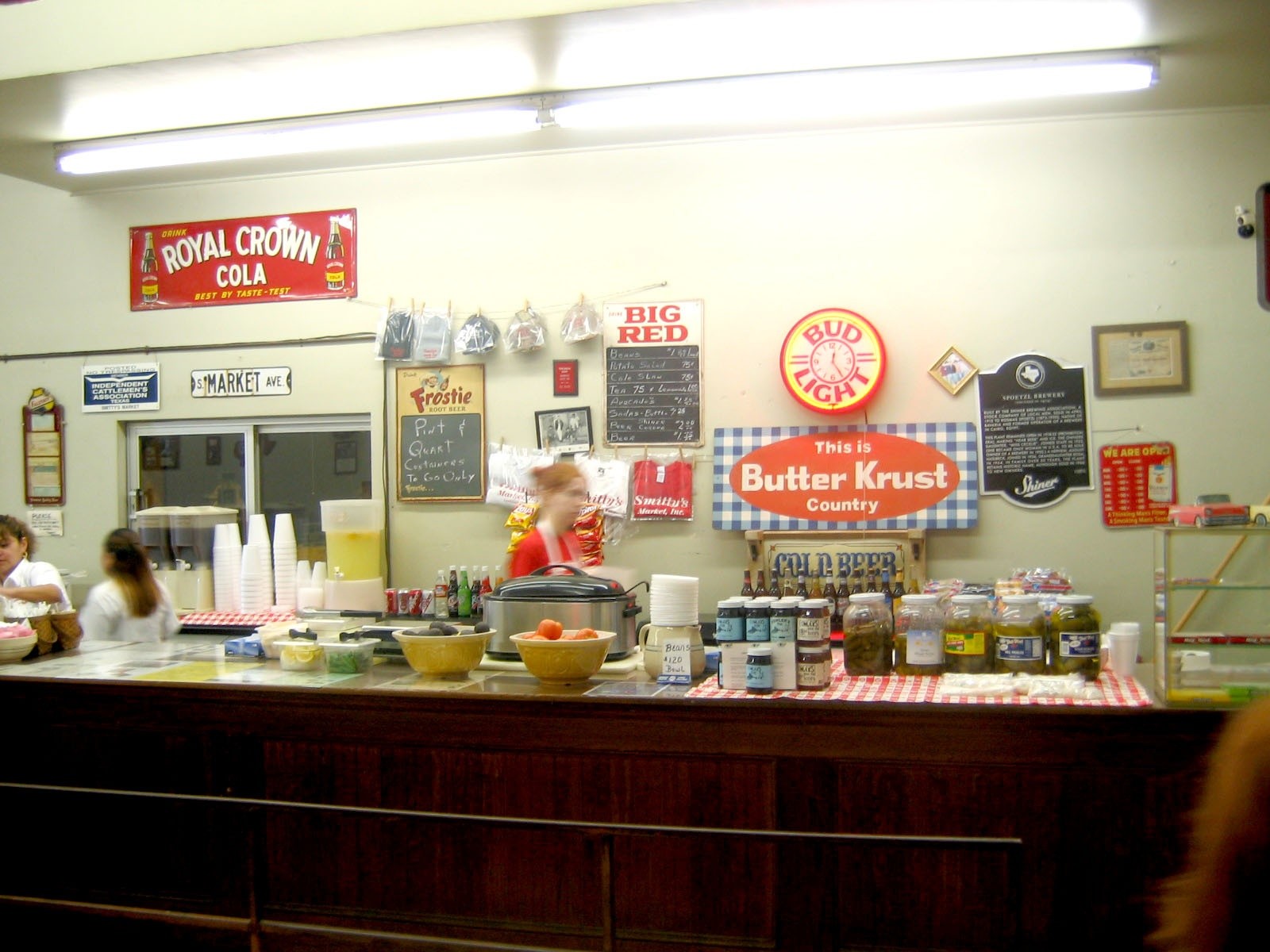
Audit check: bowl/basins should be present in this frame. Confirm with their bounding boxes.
[390,625,498,679]
[0,633,38,662]
[509,629,617,685]
[254,620,381,671]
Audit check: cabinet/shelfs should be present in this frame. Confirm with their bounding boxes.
[1152,526,1270,706]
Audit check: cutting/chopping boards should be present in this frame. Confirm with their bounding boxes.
[476,652,643,675]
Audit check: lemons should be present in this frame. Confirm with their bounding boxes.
[281,646,323,666]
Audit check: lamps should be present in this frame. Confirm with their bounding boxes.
[57,52,1159,173]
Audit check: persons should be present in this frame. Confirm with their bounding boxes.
[548,413,579,444]
[78,527,180,643]
[1148,696,1270,952]
[510,463,587,577]
[0,515,73,613]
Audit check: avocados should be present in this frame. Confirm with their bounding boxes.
[402,620,489,636]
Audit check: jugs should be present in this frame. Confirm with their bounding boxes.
[638,624,706,678]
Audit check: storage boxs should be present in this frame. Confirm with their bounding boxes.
[224,636,264,658]
[319,499,383,532]
[319,636,382,674]
[274,635,337,671]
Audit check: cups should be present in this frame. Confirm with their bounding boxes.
[213,513,328,613]
[1107,632,1141,676]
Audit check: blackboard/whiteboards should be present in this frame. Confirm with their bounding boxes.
[601,298,707,448]
[394,362,489,502]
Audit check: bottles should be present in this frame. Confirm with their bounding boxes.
[742,566,919,629]
[434,565,503,620]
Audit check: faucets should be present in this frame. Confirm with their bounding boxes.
[174,559,191,572]
[149,560,158,570]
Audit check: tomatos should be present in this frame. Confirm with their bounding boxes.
[522,619,598,641]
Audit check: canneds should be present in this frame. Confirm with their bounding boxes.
[714,595,831,695]
[385,587,435,615]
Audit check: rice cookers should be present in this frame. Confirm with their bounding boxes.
[481,565,642,660]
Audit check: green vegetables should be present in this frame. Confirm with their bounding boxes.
[325,648,371,674]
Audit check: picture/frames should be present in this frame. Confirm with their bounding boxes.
[535,406,594,457]
[334,441,359,475]
[928,345,978,396]
[1091,321,1190,397]
